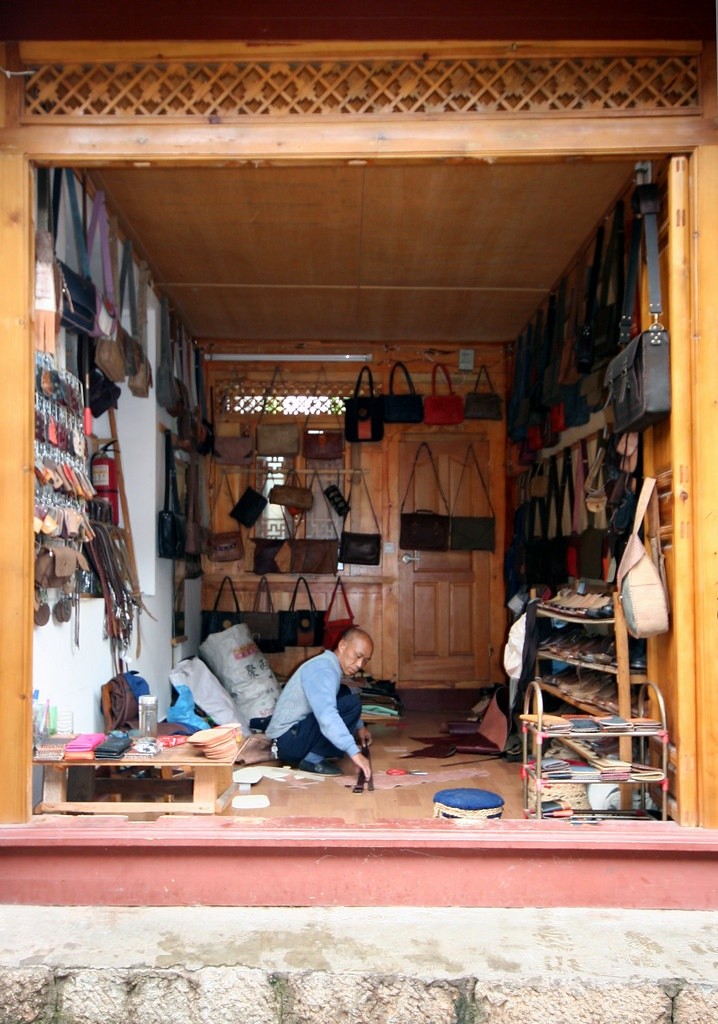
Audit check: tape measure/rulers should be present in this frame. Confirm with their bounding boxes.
[352,738,376,793]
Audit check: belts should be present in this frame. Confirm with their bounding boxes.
[84,520,144,651]
[353,740,374,793]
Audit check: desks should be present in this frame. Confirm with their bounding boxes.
[31,736,250,816]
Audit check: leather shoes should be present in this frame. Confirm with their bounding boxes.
[537,585,614,619]
[542,665,650,718]
[537,623,647,670]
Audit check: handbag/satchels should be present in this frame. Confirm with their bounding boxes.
[35,544,77,589]
[33,159,155,421]
[168,685,210,735]
[157,285,501,654]
[505,186,670,639]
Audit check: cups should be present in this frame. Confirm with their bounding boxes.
[139,695,158,738]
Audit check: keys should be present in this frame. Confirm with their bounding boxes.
[271,746,278,759]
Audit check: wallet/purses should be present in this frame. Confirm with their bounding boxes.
[34,732,162,761]
[534,757,664,783]
[520,714,663,733]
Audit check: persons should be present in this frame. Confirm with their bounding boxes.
[264,628,374,782]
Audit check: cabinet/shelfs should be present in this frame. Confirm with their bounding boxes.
[518,680,669,819]
[528,575,649,778]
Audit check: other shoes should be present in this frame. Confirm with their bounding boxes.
[480,682,504,701]
[299,758,344,776]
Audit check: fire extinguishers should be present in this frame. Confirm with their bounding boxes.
[90,440,121,526]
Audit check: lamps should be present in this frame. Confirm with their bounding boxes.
[205,353,373,362]
